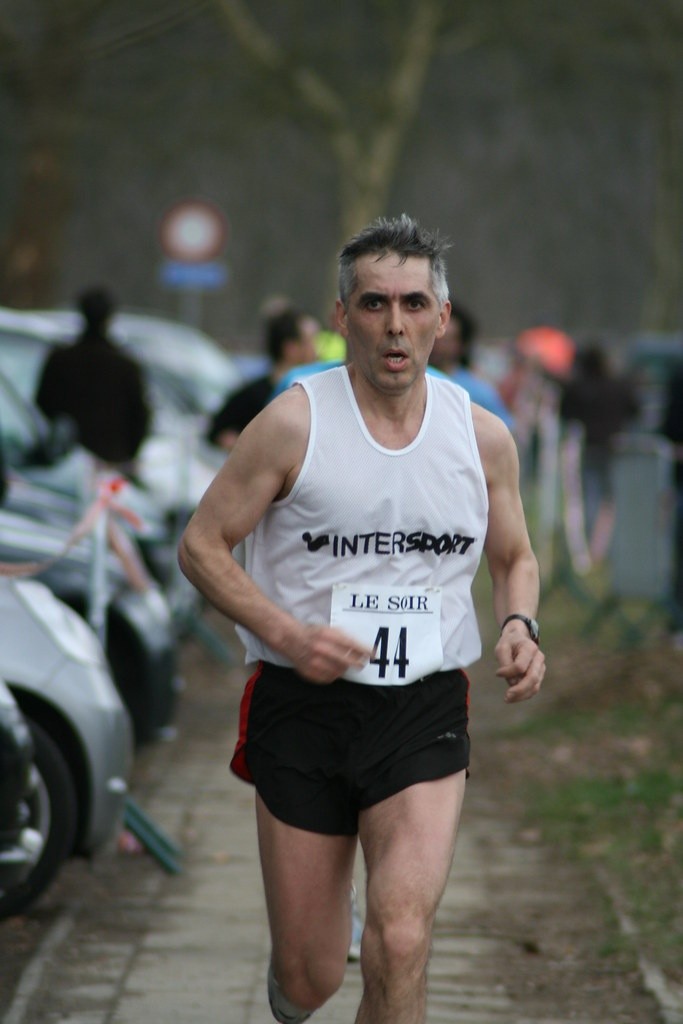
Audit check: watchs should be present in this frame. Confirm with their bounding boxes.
[502,614,540,645]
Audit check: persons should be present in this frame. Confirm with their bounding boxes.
[203,291,683,963]
[176,211,545,1024]
[34,281,151,488]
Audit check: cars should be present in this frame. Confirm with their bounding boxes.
[1,307,260,924]
